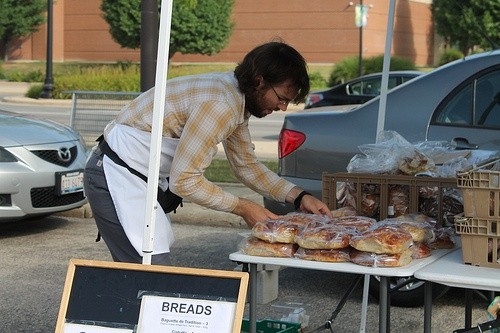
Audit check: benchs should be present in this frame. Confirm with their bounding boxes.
[67,89,149,149]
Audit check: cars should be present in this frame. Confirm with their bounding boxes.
[263,50,500,309]
[0,114,91,222]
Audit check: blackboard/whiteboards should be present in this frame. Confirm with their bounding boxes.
[54,258,249,333]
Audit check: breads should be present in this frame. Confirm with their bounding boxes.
[246,152,465,266]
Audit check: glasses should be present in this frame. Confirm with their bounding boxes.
[272,87,291,106]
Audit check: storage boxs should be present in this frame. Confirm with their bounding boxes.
[241,293,314,333]
[321,160,500,269]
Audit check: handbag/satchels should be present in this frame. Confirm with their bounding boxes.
[95,134,183,214]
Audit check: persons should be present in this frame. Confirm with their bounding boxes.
[83,42,334,268]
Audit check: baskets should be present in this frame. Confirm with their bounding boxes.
[455,213,500,270]
[241,318,303,333]
[456,156,500,219]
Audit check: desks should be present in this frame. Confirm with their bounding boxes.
[228,231,461,333]
[414,248,500,333]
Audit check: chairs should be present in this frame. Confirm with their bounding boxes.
[363,83,379,94]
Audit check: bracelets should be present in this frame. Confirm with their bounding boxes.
[294,191,312,211]
